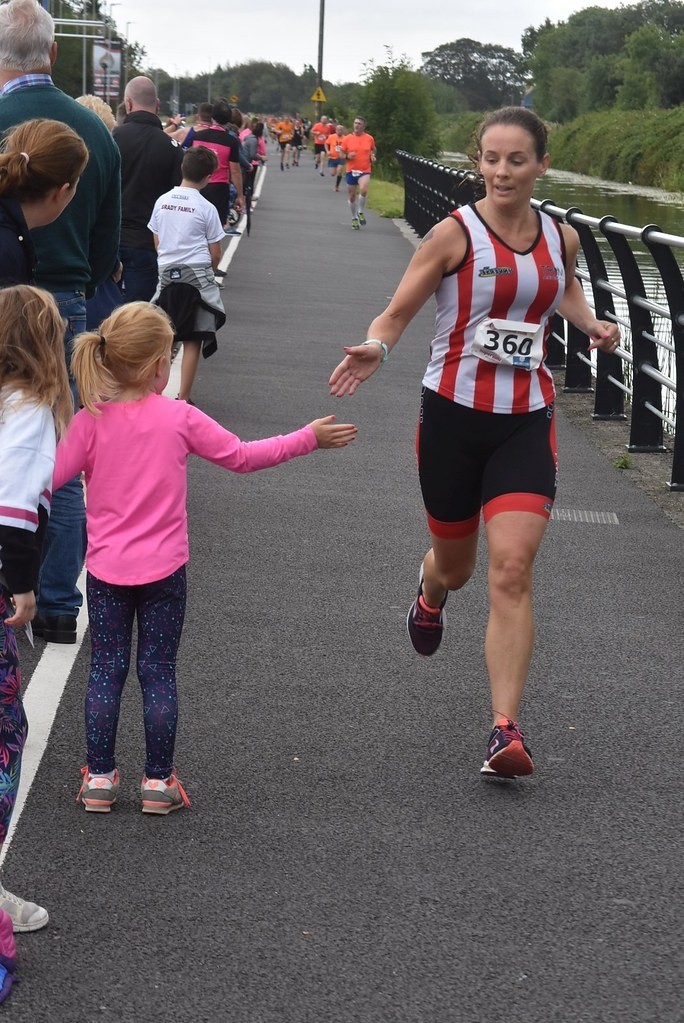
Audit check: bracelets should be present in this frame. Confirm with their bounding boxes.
[363,340,388,372]
[346,154,348,160]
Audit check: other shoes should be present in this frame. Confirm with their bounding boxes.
[334,185,339,191]
[315,164,318,169]
[319,172,324,176]
[280,162,284,170]
[174,397,196,406]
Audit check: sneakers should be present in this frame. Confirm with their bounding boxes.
[140,766,193,815]
[357,211,366,224]
[223,228,242,236]
[0,884,49,932]
[352,217,359,229]
[75,765,120,813]
[480,708,535,779]
[407,562,448,658]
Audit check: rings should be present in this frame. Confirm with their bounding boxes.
[614,341,618,348]
[611,337,616,341]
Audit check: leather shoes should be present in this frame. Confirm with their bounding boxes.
[31,613,77,643]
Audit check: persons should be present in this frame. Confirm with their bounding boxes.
[330,106,621,778]
[53,303,359,815]
[266,112,377,230]
[0,0,123,644]
[0,120,89,285]
[76,75,267,406]
[0,285,72,932]
